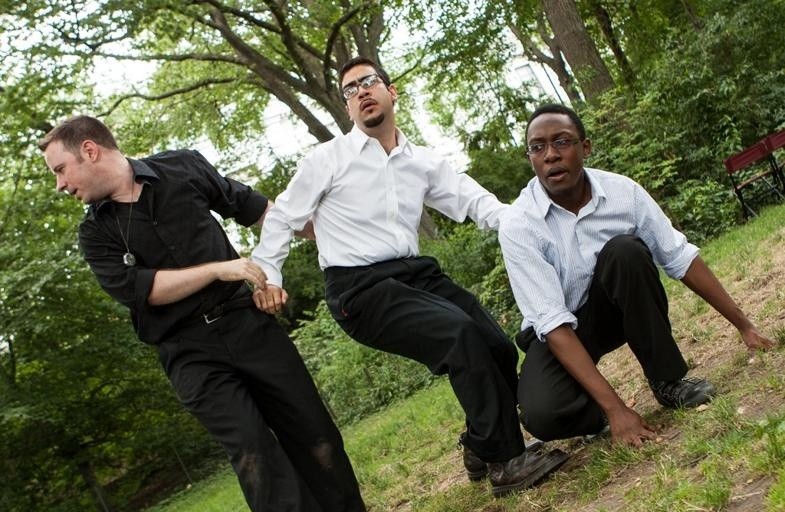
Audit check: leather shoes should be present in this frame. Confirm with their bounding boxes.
[648,376,714,407]
[457,431,569,496]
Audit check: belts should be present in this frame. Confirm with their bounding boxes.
[181,287,253,327]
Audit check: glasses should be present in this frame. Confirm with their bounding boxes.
[343,76,388,99]
[525,138,581,155]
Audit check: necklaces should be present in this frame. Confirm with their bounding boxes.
[109,175,143,266]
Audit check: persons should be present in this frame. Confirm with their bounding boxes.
[35,115,368,511]
[250,55,570,495]
[496,104,775,451]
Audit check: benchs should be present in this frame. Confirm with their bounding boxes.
[723,128,785,222]
